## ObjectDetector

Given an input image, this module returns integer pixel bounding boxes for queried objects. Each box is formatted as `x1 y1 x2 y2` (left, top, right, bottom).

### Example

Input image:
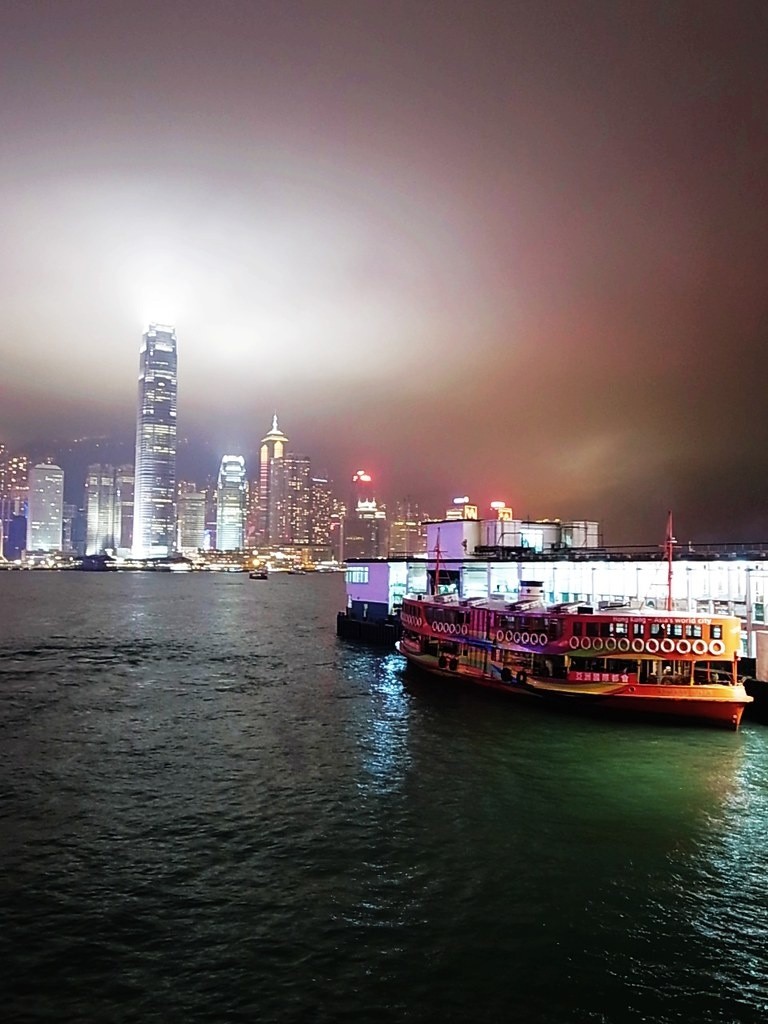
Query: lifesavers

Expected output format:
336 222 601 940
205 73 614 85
501 667 511 682
431 621 468 635
496 629 548 647
400 611 422 628
568 635 726 656
515 671 527 683
449 658 458 670
438 655 447 668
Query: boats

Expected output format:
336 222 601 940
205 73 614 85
249 570 267 580
392 509 756 733
287 567 307 575
